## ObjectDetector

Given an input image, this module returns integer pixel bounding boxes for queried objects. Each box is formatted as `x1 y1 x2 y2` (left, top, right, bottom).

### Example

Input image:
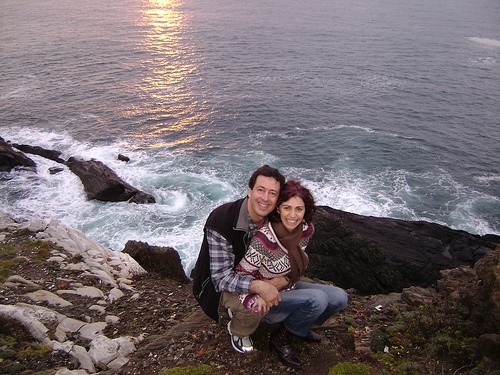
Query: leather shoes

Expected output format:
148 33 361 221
299 330 321 344
268 325 303 368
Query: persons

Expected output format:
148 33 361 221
193 165 306 354
235 181 348 368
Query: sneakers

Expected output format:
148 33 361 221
227 320 254 353
